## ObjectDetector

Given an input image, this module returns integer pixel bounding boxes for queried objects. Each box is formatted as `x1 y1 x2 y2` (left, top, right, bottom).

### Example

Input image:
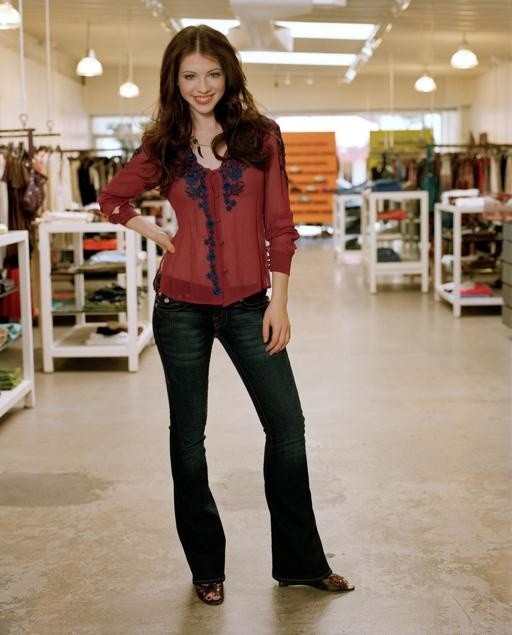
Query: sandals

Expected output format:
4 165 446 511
195 581 224 605
279 572 355 591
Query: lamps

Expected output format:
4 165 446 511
414 70 436 92
0 0 22 30
75 49 104 77
117 9 141 97
449 31 478 68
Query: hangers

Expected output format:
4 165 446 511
0 140 66 160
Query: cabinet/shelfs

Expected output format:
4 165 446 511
0 200 174 420
332 190 503 317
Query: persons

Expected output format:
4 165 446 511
96 24 356 604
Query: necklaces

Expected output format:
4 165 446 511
190 136 212 158
